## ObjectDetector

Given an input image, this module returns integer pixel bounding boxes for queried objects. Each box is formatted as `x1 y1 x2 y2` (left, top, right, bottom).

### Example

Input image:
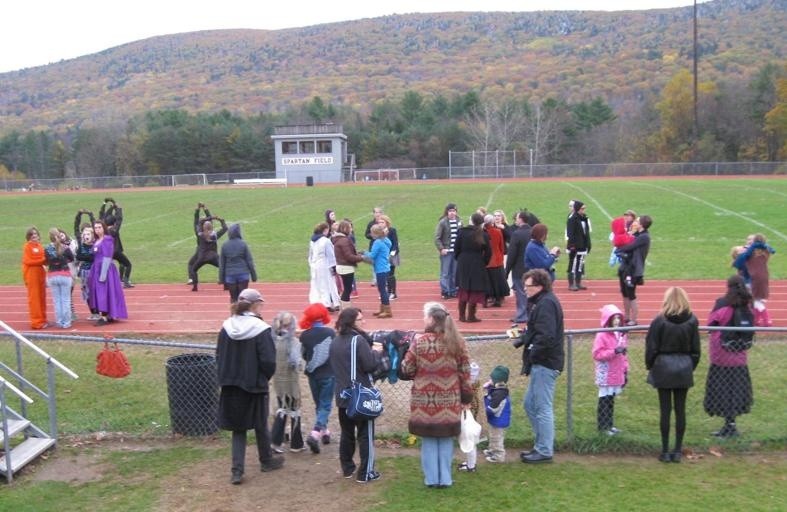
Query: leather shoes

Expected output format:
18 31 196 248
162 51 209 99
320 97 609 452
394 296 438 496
520 448 538 459
523 452 553 464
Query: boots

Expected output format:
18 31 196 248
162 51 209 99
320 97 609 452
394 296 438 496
373 304 385 316
576 271 587 290
627 297 638 326
378 304 393 318
623 297 630 323
568 272 579 291
457 300 466 322
466 303 482 322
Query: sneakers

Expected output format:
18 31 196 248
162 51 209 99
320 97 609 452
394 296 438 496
322 430 331 444
356 472 382 483
231 470 243 484
289 445 307 453
659 453 670 463
260 456 285 472
604 430 615 436
270 444 286 454
486 456 505 463
610 426 621 433
305 431 321 453
482 449 493 456
669 452 681 463
343 466 358 478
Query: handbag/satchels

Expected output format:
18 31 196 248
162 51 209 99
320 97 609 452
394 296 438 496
341 334 384 422
94 334 132 379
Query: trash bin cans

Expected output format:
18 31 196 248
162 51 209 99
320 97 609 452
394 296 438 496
164 352 221 437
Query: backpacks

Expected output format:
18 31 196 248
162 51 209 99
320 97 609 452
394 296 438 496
721 296 753 354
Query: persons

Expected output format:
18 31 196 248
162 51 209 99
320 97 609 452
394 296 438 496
483 366 512 464
592 304 630 438
369 214 398 300
360 221 392 318
74 210 97 247
328 221 344 296
566 200 592 277
492 210 512 255
22 227 52 329
504 210 533 323
481 215 510 308
341 218 358 297
58 230 80 323
88 220 127 324
523 223 561 287
703 275 755 438
434 203 463 299
215 287 285 484
187 202 216 284
330 306 383 483
365 208 384 286
610 216 636 289
644 287 701 463
330 222 364 308
454 213 492 323
520 267 565 464
745 234 772 327
103 207 134 288
218 223 257 305
614 215 653 327
43 228 73 329
270 310 307 454
190 216 228 292
104 199 126 283
618 209 638 237
458 362 480 474
509 213 518 242
400 301 473 489
732 246 752 296
307 222 341 313
298 303 337 455
477 206 488 229
75 228 103 321
567 201 589 292
325 209 336 238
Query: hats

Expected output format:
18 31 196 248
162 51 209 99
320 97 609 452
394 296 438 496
490 365 510 383
574 200 583 212
237 287 264 304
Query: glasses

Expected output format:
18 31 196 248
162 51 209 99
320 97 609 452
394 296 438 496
524 283 537 288
